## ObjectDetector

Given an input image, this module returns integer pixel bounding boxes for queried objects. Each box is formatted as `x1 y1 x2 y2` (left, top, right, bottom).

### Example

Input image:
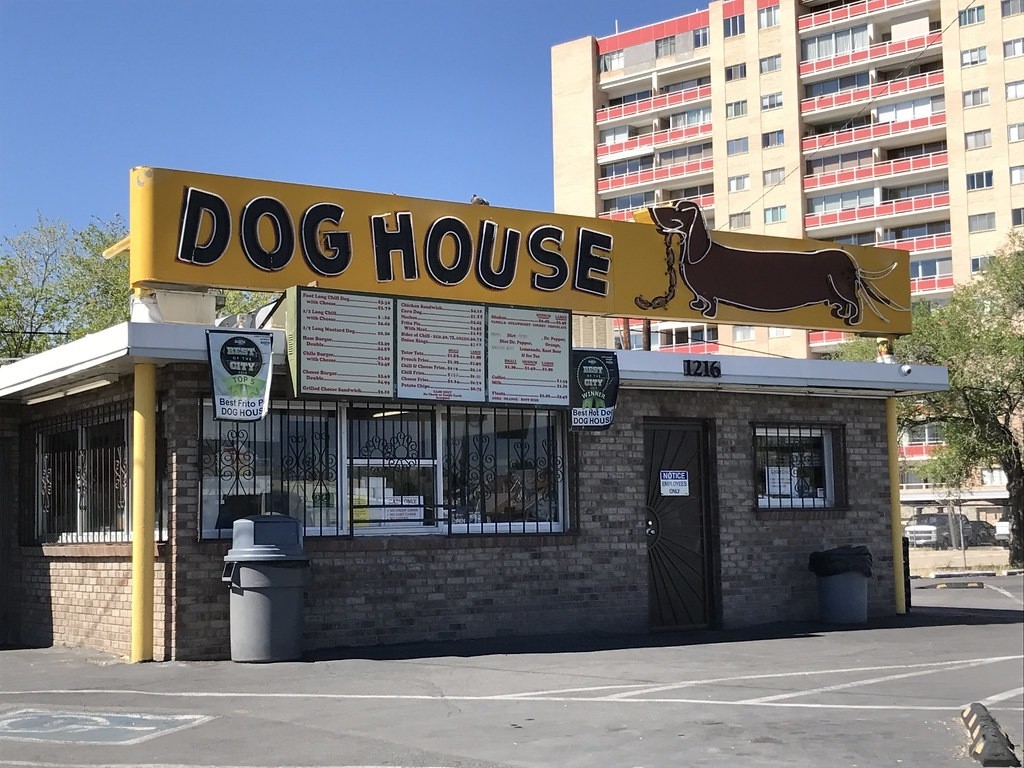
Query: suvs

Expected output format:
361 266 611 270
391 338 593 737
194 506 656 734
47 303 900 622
904 512 972 551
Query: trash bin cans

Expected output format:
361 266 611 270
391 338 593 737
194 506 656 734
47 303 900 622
223 512 312 664
808 545 875 625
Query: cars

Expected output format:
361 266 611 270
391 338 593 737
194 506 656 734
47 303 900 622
995 518 1013 546
967 521 999 546
